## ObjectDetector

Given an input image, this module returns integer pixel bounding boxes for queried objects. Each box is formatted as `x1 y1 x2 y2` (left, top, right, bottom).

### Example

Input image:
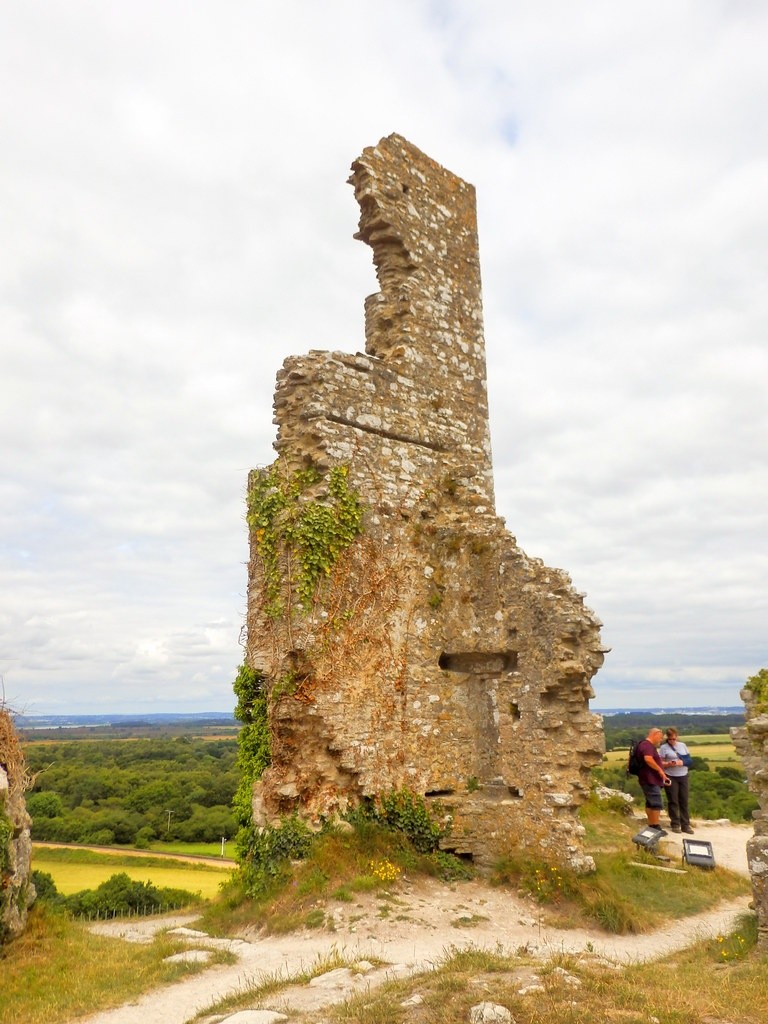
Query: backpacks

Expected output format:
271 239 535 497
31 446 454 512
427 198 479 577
626 738 649 779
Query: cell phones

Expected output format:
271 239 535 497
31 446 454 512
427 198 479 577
672 761 676 765
663 780 672 785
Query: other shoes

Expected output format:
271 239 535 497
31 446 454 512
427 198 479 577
672 825 682 833
681 824 694 834
650 824 668 836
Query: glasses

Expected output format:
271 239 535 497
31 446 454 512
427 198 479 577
669 738 676 740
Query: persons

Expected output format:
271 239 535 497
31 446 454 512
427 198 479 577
658 728 694 834
628 728 673 836
